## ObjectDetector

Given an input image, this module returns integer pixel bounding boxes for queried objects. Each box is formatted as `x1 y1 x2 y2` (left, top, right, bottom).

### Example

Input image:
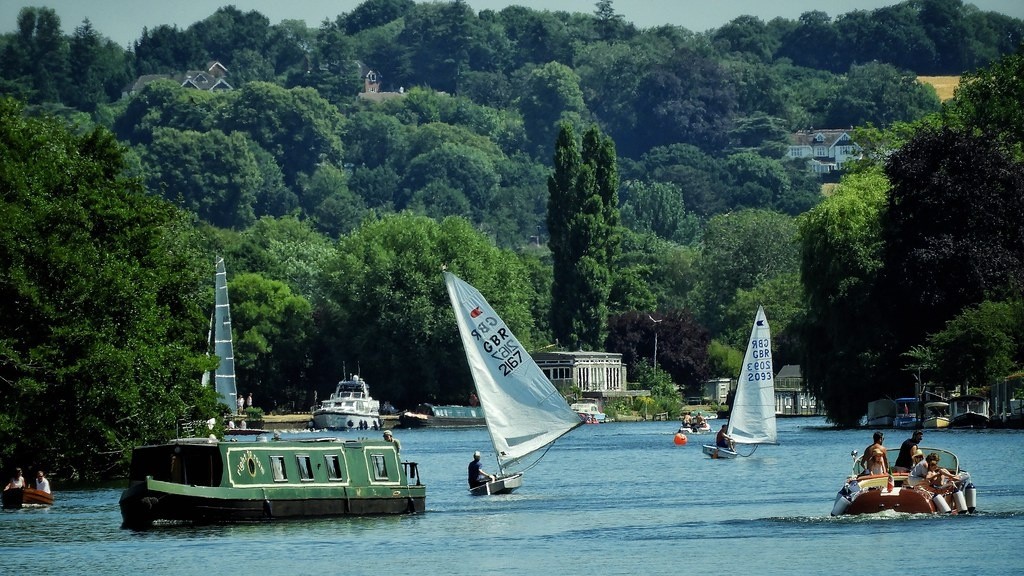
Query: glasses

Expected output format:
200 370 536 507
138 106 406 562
915 456 923 458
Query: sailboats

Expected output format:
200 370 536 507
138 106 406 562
701 305 778 460
441 263 588 495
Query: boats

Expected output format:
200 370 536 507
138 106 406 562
892 397 922 427
866 399 895 427
118 433 427 528
831 446 977 516
311 360 382 430
946 394 991 428
921 402 951 429
570 401 606 423
398 404 488 427
1 486 55 510
679 419 711 434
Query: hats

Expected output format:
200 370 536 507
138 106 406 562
872 449 883 457
383 430 392 437
911 450 926 460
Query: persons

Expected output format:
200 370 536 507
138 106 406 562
682 412 692 427
912 449 927 463
870 448 886 476
584 414 597 421
900 452 941 487
246 392 253 407
229 417 235 429
382 430 400 477
36 470 51 495
373 420 378 431
237 395 245 415
860 432 889 473
4 468 25 491
925 461 962 487
716 424 736 452
895 430 924 472
239 419 246 429
468 450 495 489
359 419 365 430
695 411 703 425
363 420 367 430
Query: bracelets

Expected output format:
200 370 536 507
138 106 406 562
486 474 491 477
